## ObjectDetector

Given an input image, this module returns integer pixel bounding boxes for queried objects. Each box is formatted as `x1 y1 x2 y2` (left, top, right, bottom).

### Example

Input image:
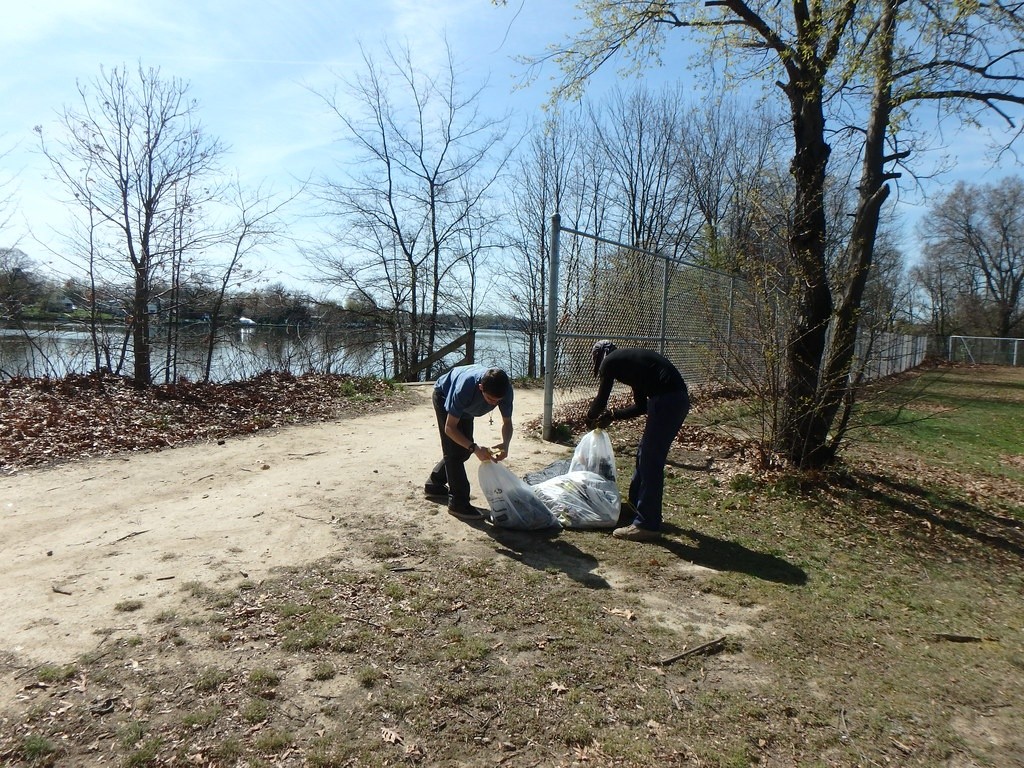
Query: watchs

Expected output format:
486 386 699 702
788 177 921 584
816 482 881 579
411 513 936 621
469 443 477 454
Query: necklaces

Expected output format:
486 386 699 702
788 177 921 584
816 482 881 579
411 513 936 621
488 410 493 425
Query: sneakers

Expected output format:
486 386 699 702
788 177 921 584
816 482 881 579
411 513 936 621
612 523 661 542
621 503 638 519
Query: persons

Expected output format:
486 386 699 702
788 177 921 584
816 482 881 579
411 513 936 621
586 340 690 540
423 364 514 519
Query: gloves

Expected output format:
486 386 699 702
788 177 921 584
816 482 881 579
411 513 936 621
586 415 596 430
597 408 615 429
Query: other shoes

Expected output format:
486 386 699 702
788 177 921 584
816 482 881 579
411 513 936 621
447 501 484 520
424 483 448 498
